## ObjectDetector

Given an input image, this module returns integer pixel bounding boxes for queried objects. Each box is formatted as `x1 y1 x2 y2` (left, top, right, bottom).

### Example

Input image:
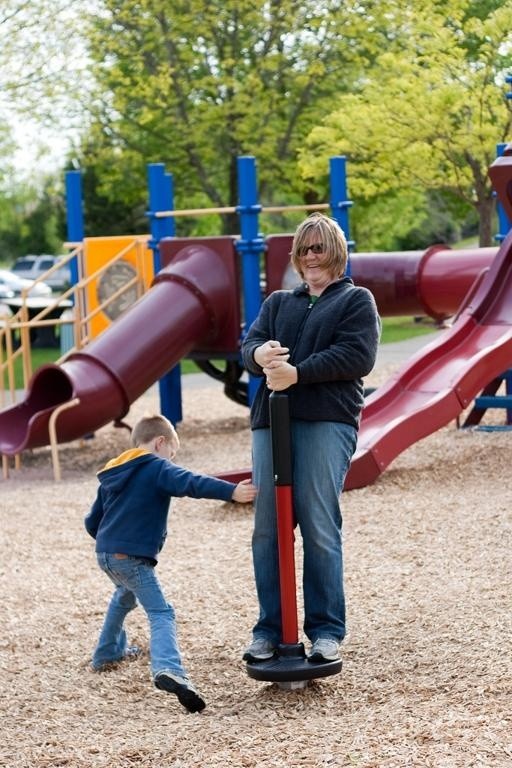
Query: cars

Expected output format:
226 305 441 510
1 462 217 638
0 256 72 348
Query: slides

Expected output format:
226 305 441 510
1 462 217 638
0 244 235 457
208 227 511 495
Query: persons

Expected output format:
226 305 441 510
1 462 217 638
241 211 381 663
85 414 258 713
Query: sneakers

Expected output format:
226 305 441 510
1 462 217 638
91 646 143 672
307 637 341 661
242 638 278 660
155 672 206 713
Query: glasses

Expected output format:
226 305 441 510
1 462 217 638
297 243 324 256
